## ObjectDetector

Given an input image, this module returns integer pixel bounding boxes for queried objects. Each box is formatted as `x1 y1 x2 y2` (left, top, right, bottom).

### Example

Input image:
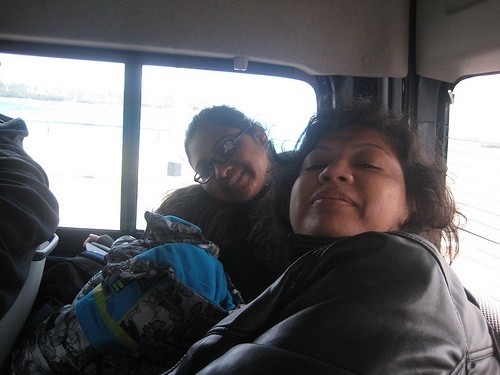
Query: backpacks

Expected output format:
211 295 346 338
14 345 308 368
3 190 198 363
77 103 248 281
7 209 247 375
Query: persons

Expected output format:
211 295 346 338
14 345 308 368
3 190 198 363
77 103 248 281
82 106 306 305
0 114 59 322
166 91 500 375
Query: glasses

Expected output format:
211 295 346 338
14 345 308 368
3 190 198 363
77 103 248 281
193 125 252 185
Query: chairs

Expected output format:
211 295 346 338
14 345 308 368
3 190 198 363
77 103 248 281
0 232 58 364
